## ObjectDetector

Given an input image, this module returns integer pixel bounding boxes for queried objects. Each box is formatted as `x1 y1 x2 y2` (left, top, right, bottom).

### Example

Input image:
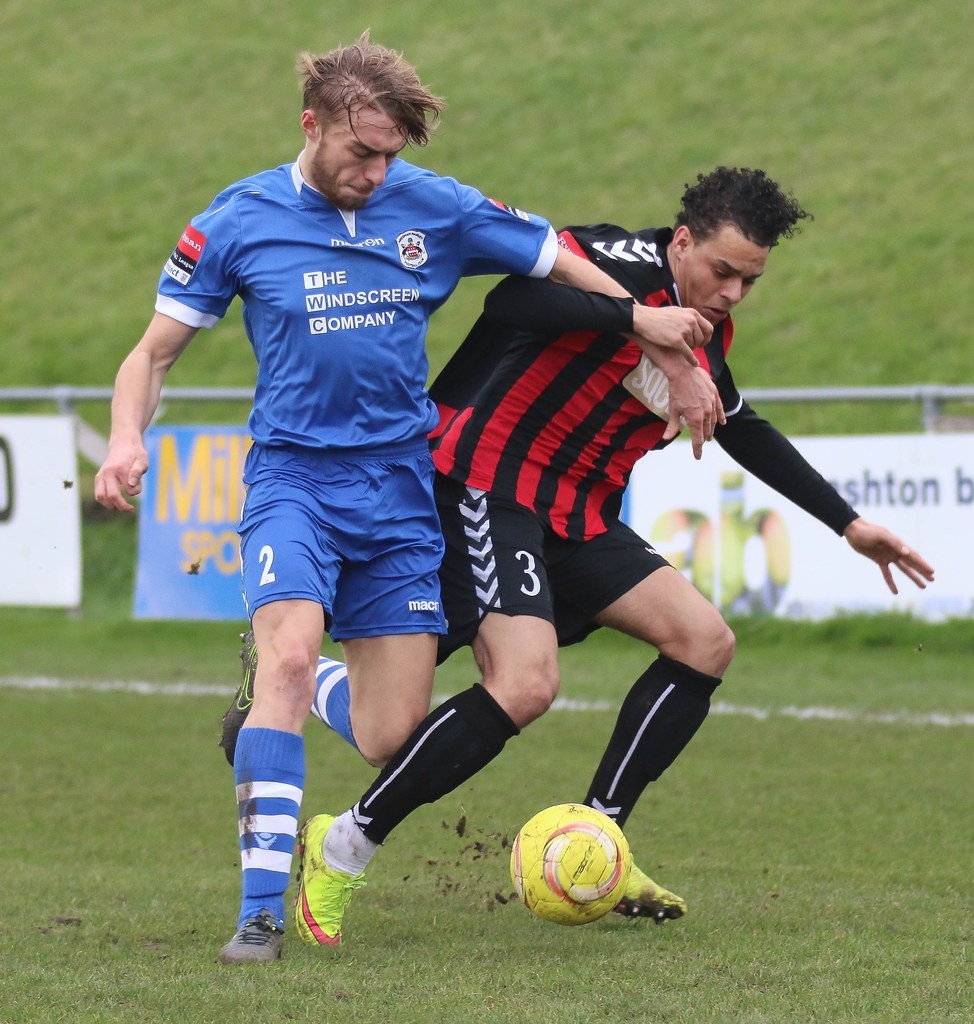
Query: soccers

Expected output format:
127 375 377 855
509 803 632 927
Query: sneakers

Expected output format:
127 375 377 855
295 814 366 947
614 861 688 924
217 630 257 766
219 908 286 965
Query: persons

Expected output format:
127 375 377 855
292 167 939 953
92 40 728 971
